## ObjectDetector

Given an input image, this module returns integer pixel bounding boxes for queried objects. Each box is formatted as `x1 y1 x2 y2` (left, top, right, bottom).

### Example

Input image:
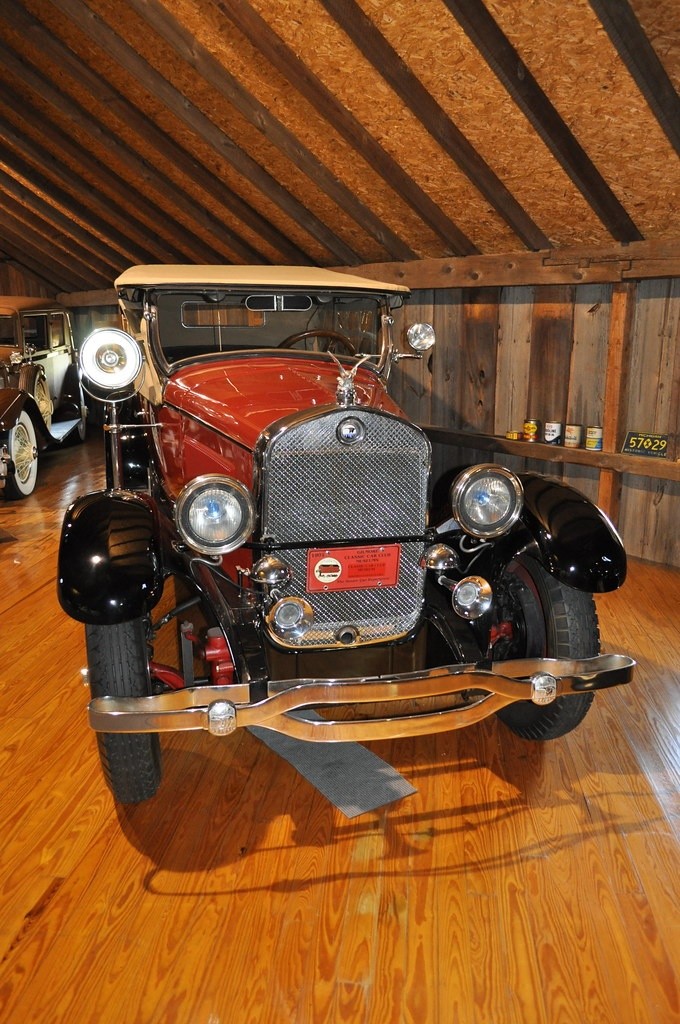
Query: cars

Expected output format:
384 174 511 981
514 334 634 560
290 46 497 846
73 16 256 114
0 296 90 500
57 263 639 805
